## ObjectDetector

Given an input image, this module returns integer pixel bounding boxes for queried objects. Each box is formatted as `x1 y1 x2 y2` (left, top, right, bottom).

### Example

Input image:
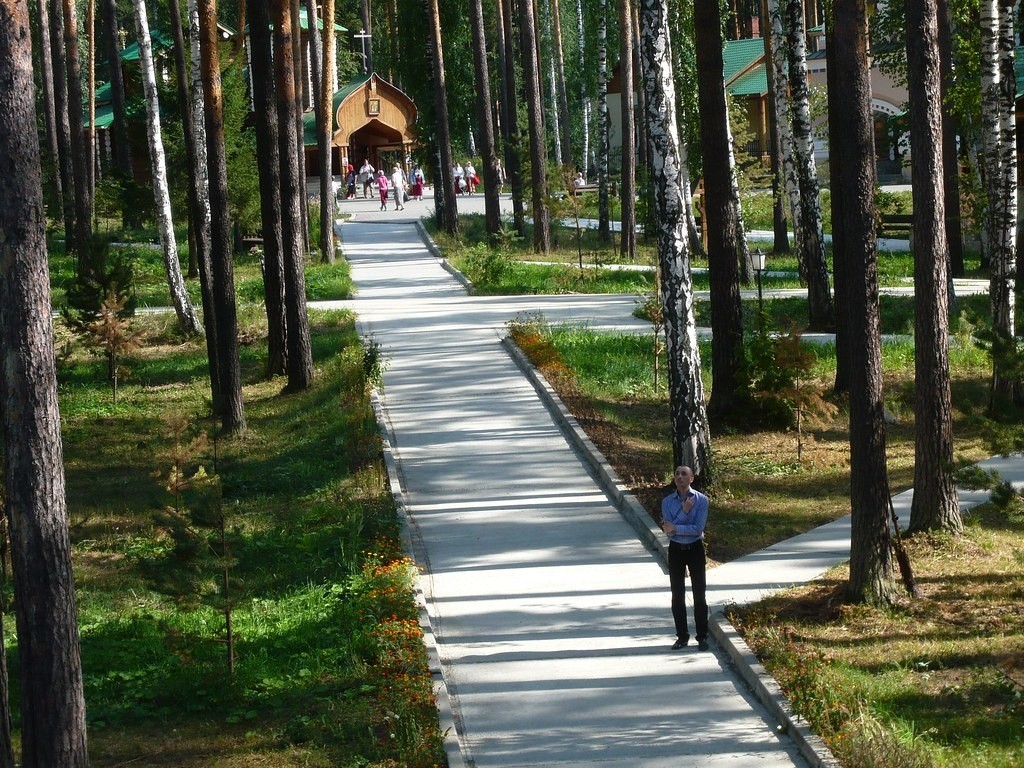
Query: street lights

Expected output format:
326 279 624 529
750 244 769 339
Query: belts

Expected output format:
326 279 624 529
670 538 702 551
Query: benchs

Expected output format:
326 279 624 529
562 182 617 201
876 213 914 240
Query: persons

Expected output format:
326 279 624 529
332 176 340 212
593 173 601 184
359 159 376 198
561 172 586 200
661 464 710 650
464 161 476 196
452 162 465 196
375 170 389 211
346 165 357 199
494 159 506 195
391 162 407 211
411 164 425 201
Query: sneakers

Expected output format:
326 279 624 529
671 638 688 649
699 638 709 651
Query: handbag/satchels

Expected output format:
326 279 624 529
385 191 388 198
458 177 467 189
472 175 480 184
404 192 410 202
367 177 374 183
